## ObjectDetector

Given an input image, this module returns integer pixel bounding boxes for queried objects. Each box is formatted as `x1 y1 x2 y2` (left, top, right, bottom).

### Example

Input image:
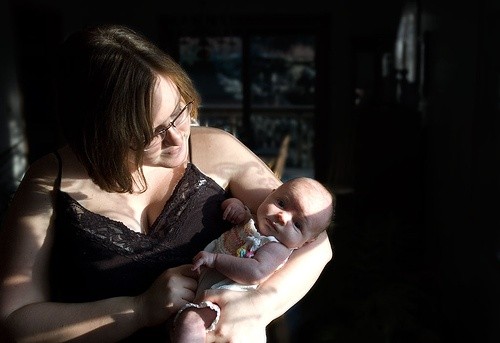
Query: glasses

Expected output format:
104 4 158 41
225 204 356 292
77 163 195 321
128 82 196 152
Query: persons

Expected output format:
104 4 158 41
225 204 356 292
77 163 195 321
0 27 333 343
170 177 336 343
